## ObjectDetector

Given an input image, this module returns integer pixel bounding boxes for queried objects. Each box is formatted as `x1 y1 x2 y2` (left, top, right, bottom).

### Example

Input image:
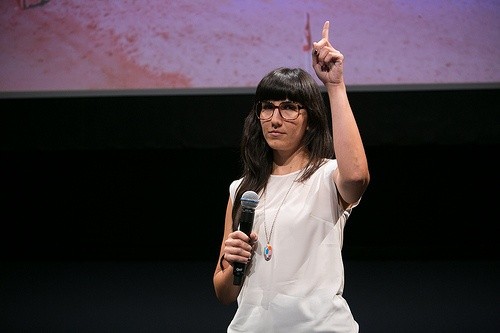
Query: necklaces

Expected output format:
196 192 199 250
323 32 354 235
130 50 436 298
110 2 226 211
263 171 301 261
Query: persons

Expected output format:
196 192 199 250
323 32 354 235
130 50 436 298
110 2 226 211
213 21 371 333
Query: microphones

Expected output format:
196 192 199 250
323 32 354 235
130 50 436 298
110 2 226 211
233 191 260 286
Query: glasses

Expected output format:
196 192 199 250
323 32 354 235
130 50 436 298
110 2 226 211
256 100 305 120
